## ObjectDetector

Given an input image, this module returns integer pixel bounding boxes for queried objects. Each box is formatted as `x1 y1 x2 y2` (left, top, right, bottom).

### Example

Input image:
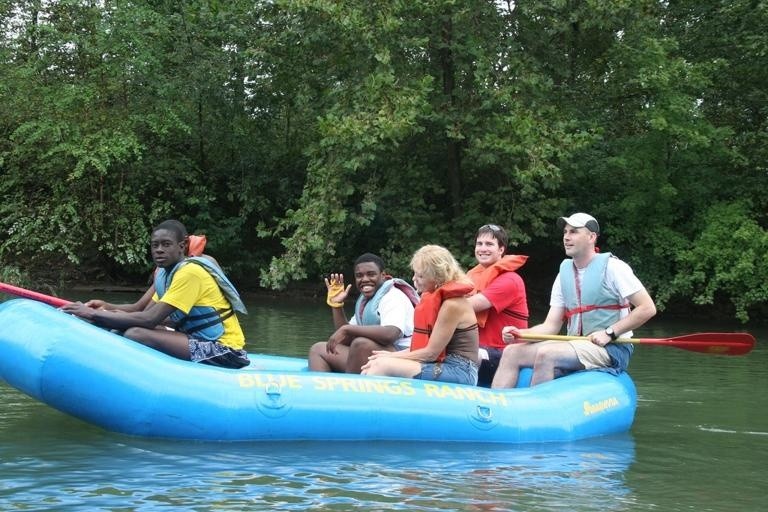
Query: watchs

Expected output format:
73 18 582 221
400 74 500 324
604 325 618 341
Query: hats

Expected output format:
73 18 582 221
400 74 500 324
557 212 600 236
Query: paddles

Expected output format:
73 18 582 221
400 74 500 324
507 333 754 354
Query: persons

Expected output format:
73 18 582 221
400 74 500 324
61 219 251 369
307 252 421 374
360 243 480 385
414 223 530 386
490 212 657 390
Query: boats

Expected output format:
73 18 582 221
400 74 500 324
0 297 638 446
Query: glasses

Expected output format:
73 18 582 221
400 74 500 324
479 225 501 232
